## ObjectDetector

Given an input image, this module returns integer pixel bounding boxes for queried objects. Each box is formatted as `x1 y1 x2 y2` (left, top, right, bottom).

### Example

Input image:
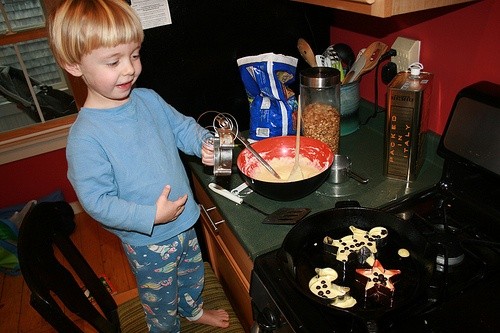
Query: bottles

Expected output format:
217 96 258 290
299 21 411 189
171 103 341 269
298 66 341 155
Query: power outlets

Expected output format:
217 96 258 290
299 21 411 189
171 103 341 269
391 35 421 73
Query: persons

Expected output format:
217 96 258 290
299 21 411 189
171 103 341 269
46 0 229 333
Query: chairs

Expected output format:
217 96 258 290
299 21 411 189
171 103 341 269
16 199 246 333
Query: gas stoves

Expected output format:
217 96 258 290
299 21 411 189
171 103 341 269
248 82 500 333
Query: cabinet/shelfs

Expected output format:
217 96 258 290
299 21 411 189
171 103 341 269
192 171 252 333
293 0 473 17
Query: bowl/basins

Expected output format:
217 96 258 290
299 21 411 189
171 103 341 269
236 135 335 202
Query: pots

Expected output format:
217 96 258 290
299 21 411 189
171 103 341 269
279 200 438 324
